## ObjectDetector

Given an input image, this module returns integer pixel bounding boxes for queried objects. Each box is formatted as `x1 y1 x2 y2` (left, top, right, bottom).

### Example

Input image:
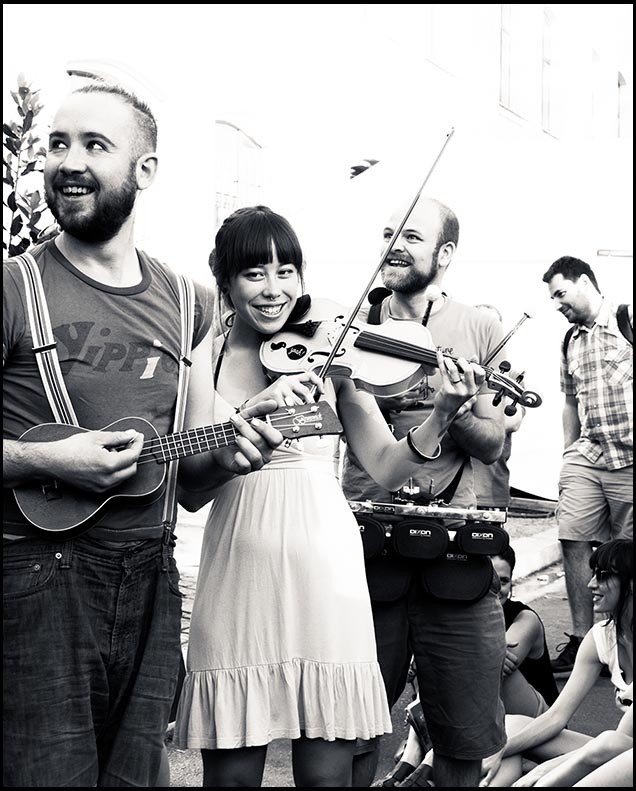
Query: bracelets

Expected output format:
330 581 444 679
407 425 442 462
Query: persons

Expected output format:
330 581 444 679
0 83 283 788
333 198 633 788
178 206 478 787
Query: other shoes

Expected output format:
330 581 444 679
550 632 585 679
367 761 436 789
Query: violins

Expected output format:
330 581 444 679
258 293 543 416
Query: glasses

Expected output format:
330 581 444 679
592 566 617 581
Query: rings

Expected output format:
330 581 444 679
453 378 462 383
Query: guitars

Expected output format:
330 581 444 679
12 400 344 533
